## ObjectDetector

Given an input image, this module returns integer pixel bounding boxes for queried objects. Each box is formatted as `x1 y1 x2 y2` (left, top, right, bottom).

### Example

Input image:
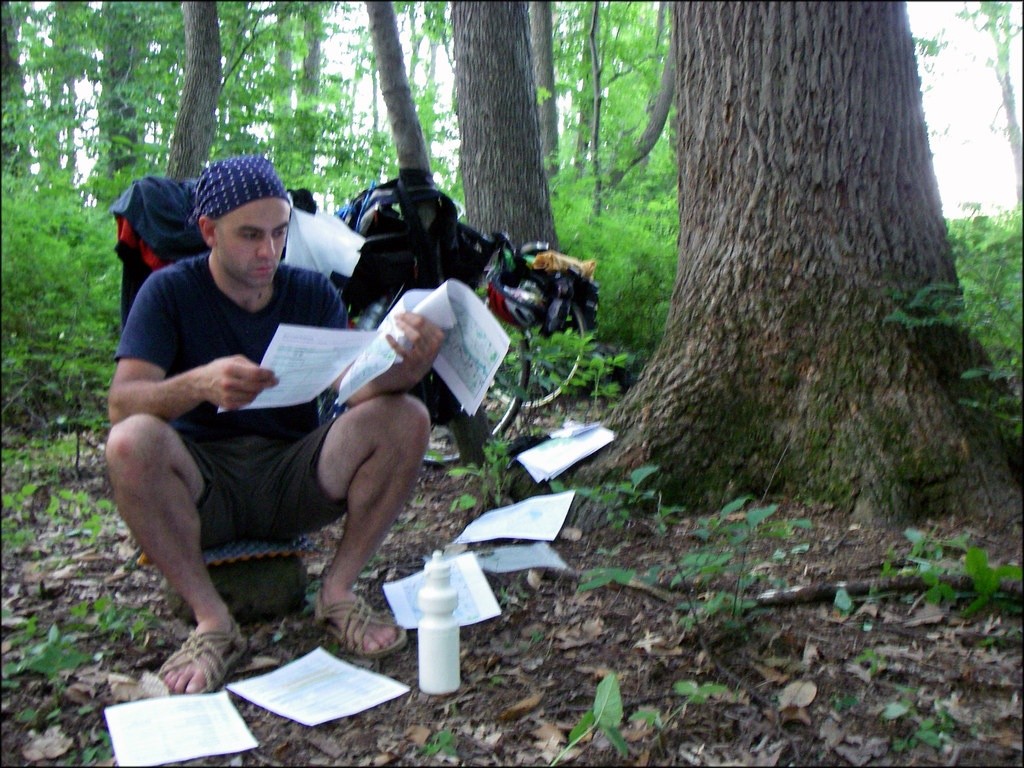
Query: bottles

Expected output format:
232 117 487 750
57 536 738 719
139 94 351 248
355 296 386 330
449 400 493 467
416 548 460 694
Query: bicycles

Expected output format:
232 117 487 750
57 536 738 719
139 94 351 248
315 231 590 468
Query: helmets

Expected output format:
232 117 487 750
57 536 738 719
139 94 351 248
486 265 543 328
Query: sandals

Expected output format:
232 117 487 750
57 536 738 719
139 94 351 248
158 615 241 695
313 584 407 658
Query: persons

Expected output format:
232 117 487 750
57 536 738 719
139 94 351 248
104 156 448 699
